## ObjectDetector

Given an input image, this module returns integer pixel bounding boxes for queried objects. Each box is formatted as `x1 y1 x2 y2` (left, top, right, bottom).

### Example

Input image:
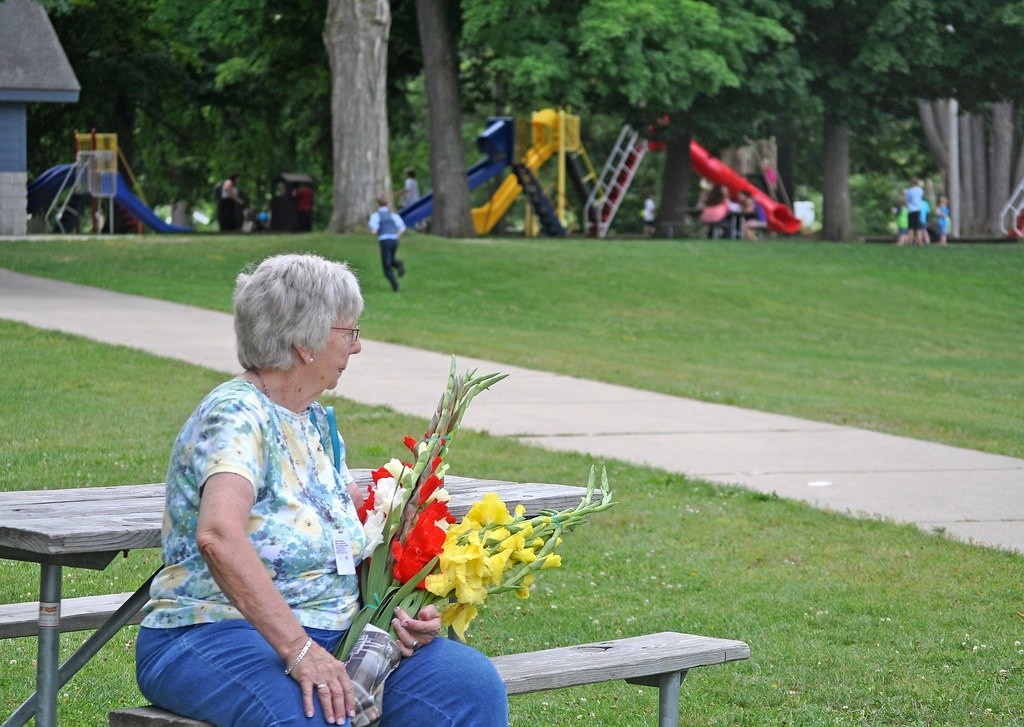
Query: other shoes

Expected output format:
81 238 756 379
395 259 404 277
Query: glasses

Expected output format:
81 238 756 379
330 324 361 342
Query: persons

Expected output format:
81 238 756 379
699 183 768 238
367 196 406 291
893 176 953 246
216 172 249 231
644 194 657 235
394 169 426 232
133 253 508 727
292 183 314 232
244 204 269 231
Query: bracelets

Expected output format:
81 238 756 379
284 637 312 674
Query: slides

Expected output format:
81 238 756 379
472 144 553 235
398 159 506 228
655 126 802 233
103 167 191 234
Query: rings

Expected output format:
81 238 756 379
410 640 418 649
317 684 326 689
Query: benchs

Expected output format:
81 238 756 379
0 590 152 642
702 220 765 231
109 631 751 727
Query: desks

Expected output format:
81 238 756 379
674 209 751 239
0 470 607 727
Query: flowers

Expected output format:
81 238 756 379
350 364 611 645
